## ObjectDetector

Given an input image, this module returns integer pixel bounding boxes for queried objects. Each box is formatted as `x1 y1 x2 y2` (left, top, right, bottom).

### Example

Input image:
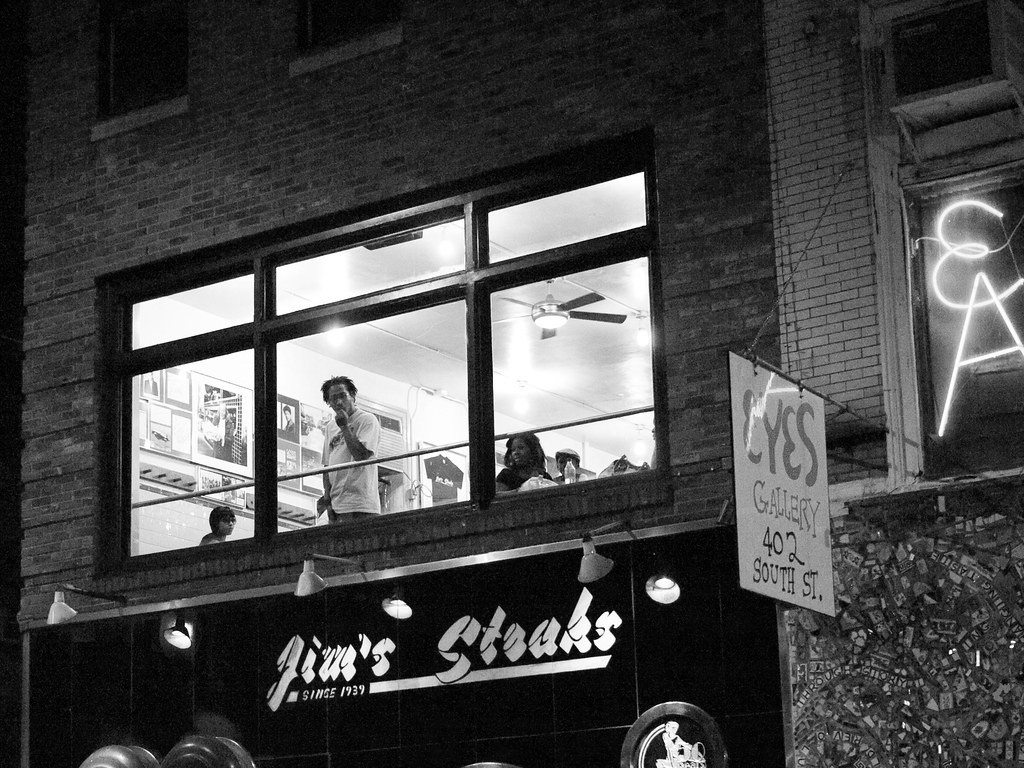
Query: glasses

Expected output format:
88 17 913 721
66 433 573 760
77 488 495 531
330 391 351 401
222 516 237 525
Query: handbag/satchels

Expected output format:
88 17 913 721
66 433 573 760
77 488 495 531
598 455 650 478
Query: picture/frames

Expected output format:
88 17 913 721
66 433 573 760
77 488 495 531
138 368 332 510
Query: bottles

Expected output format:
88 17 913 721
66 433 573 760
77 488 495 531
564 458 576 484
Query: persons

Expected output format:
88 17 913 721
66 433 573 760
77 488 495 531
199 505 237 546
495 431 553 494
281 406 295 433
551 448 587 484
144 371 159 396
650 416 657 470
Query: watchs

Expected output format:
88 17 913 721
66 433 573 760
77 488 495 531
319 374 381 524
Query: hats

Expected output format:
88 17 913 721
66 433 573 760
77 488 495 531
555 448 580 461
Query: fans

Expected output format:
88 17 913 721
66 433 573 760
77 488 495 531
489 292 628 340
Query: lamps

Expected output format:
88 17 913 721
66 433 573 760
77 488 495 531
380 582 414 620
46 583 129 626
162 608 192 649
293 551 376 597
577 517 631 583
645 550 685 606
